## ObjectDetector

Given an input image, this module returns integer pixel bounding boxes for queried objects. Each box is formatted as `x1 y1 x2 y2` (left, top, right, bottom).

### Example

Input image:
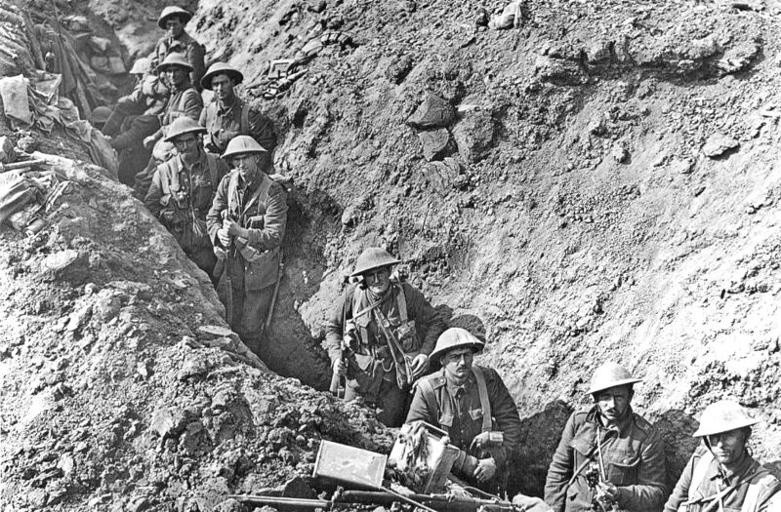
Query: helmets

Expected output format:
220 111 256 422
157 6 192 30
582 360 644 396
428 327 486 361
218 135 269 160
692 398 761 442
348 247 403 279
200 62 244 90
129 58 155 75
162 117 207 143
155 52 195 75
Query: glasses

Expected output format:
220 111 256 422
709 432 748 445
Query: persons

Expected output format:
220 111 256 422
664 396 781 512
539 359 667 511
403 325 524 495
319 244 446 427
155 3 205 79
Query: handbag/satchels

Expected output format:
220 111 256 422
477 448 511 491
396 350 440 389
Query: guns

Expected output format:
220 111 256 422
230 485 523 511
